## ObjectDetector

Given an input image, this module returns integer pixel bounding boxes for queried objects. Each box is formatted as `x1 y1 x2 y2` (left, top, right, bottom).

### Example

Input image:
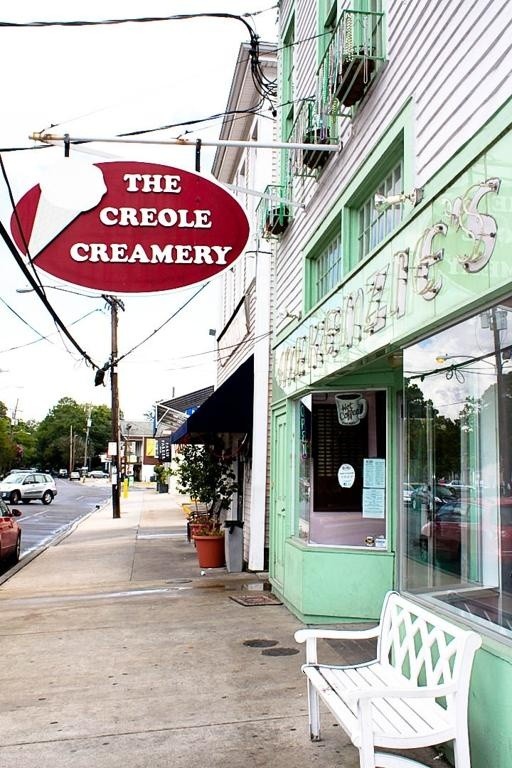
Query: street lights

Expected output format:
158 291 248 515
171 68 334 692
435 355 512 479
16 285 122 518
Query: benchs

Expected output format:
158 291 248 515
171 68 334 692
294 593 484 765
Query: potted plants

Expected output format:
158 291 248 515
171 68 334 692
153 437 241 567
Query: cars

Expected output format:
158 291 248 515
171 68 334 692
88 470 110 479
403 479 512 563
0 496 22 563
0 468 58 505
59 469 80 481
